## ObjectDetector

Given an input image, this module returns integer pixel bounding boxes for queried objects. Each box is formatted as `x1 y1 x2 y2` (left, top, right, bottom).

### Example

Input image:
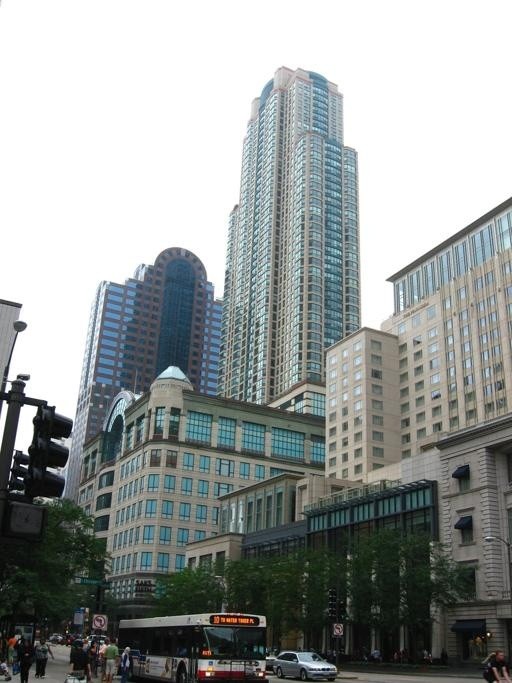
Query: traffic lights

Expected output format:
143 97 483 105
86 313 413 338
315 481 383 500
24 403 75 498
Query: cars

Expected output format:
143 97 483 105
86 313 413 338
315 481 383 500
272 650 338 682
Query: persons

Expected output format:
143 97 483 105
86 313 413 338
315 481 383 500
265 647 433 664
67 639 131 683
483 650 512 683
0 631 54 683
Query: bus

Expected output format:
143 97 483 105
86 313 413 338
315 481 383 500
116 611 270 683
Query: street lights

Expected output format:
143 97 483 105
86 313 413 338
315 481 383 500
484 536 512 595
